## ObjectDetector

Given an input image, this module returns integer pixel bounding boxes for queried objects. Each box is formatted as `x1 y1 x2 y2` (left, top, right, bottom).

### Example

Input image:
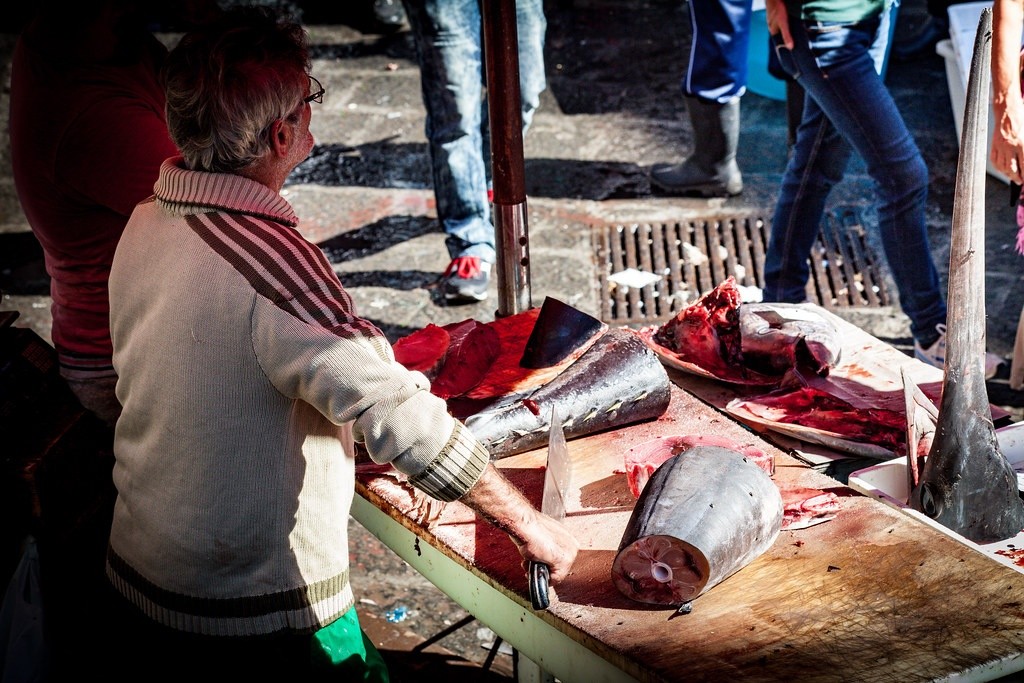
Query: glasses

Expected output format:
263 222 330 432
299 75 324 107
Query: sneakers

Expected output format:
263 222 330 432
914 323 1008 382
442 255 492 301
486 189 496 227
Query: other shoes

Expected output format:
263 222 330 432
983 334 1015 360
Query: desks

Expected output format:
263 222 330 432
350 370 1024 682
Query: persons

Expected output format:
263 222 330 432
650 0 1023 389
97 8 579 683
404 0 548 304
8 1 185 436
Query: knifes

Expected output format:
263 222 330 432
528 404 573 611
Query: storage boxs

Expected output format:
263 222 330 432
849 418 1024 575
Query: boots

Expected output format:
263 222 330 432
785 80 805 158
647 97 742 195
